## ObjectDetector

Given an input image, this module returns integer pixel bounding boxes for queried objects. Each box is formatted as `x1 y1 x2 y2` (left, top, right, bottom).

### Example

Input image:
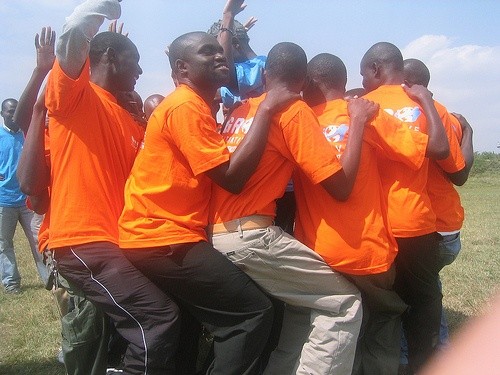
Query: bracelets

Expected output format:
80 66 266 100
217 25 234 37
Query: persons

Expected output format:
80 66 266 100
143 94 166 120
293 52 450 374
44 1 184 374
117 33 275 375
119 92 144 121
346 88 368 100
207 0 299 373
397 58 469 374
16 84 117 373
1 98 51 290
209 41 380 375
17 26 56 151
355 42 475 374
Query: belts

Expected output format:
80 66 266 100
205 215 275 235
